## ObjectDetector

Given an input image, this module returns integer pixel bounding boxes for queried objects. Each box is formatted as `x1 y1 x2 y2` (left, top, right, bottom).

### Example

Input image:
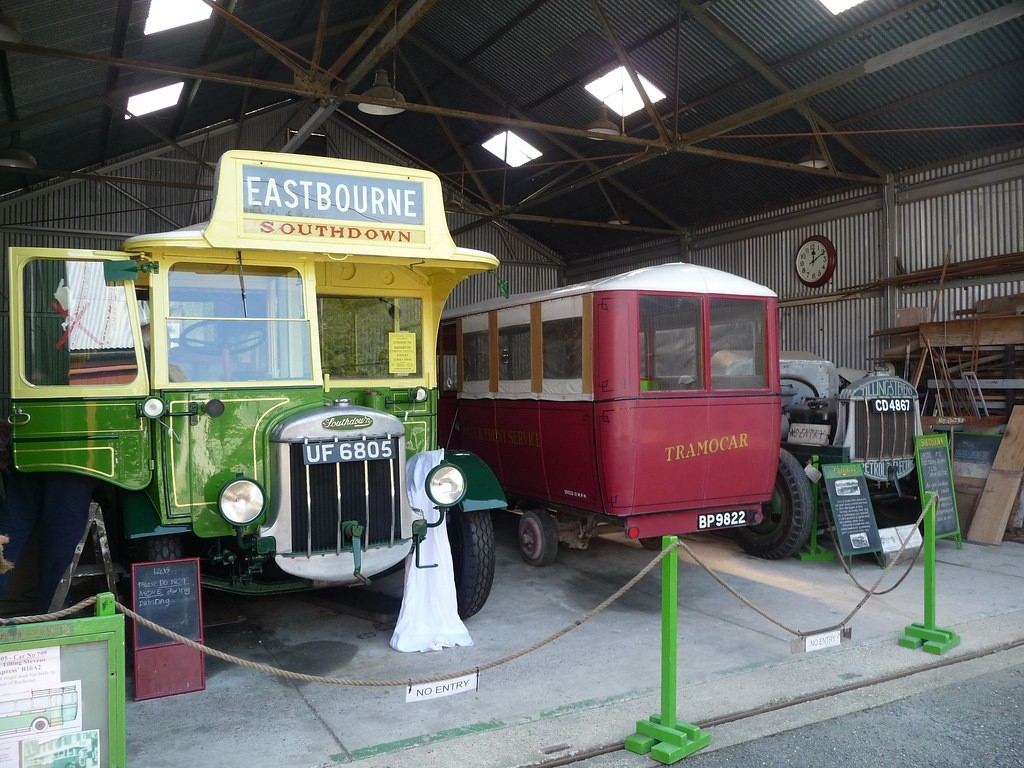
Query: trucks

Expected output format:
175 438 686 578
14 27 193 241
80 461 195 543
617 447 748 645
690 348 926 562
2 149 511 622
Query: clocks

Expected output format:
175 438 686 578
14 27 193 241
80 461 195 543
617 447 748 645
794 235 837 288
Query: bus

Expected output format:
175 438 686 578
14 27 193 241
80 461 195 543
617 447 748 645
437 259 783 568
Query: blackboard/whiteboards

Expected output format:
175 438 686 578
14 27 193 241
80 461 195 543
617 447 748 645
822 477 890 556
914 448 967 538
131 557 204 654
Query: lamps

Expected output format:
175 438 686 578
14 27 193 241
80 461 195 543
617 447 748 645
0 147 38 169
357 43 405 116
798 138 828 169
583 103 620 141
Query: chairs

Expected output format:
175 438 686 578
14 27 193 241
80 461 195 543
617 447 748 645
170 346 238 382
866 307 929 383
